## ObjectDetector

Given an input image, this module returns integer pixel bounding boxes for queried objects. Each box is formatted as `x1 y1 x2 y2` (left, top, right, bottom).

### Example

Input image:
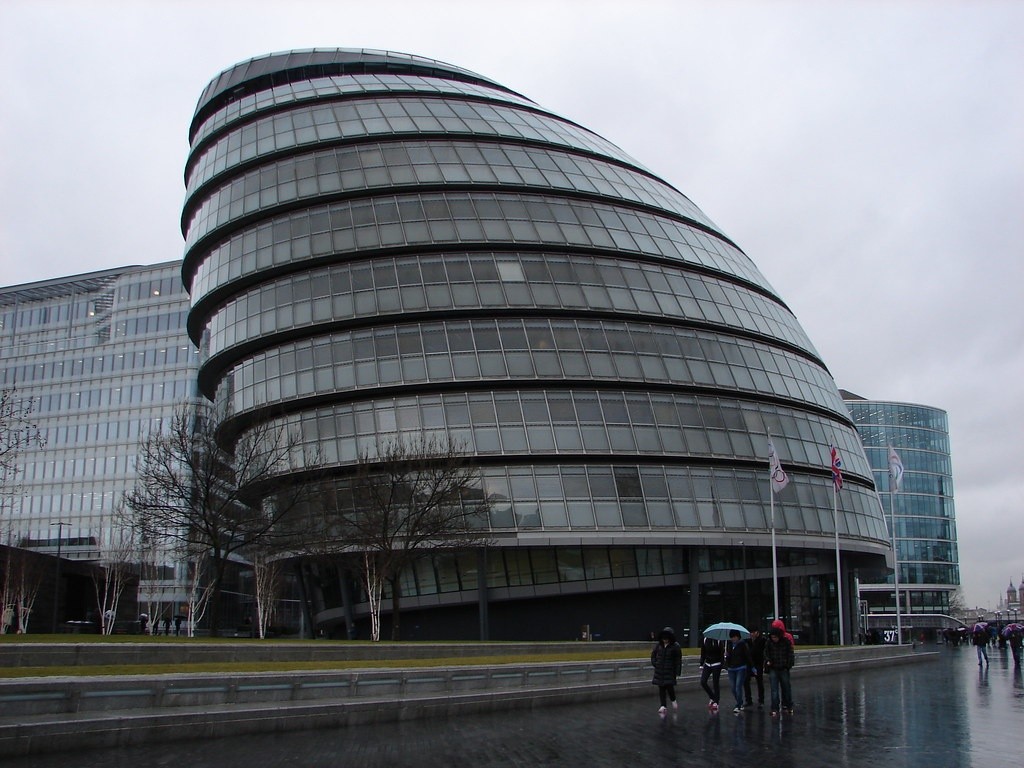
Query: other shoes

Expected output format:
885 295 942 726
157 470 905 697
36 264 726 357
740 706 744 710
772 711 776 717
788 708 793 713
707 699 714 706
733 707 740 712
711 702 719 713
671 699 677 712
658 705 668 713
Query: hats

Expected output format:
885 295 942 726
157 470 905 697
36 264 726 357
748 623 761 632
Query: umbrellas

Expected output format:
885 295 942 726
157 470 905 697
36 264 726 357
704 622 750 660
970 622 987 633
1001 623 1024 638
174 614 186 618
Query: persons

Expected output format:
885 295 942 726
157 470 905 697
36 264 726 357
652 628 683 713
140 614 182 637
859 622 1024 665
699 620 797 717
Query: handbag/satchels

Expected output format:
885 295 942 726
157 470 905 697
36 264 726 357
651 650 657 667
763 660 769 674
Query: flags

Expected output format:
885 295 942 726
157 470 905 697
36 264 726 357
888 447 904 494
768 435 789 494
830 446 843 491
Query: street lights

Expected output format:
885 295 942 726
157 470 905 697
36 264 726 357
50 521 73 635
